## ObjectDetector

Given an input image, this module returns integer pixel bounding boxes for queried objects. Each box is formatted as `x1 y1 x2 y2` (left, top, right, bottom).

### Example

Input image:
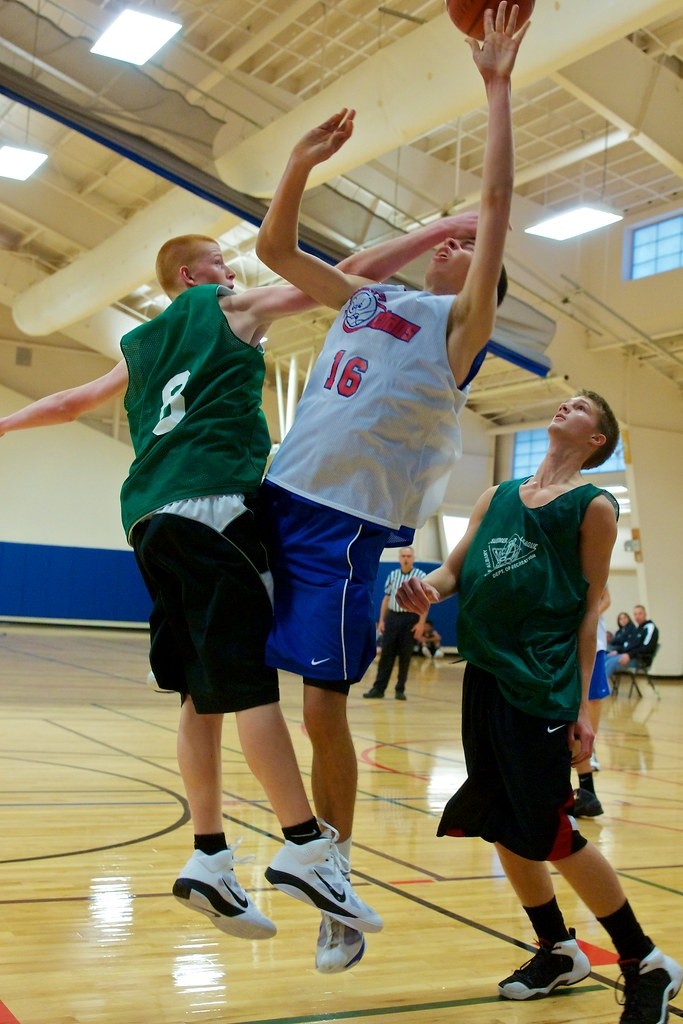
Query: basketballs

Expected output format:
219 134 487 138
445 0 535 41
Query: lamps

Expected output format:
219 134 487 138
0 0 47 181
90 0 182 66
523 119 625 242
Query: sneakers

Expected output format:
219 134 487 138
566 794 604 818
316 910 365 973
615 938 683 1024
171 837 276 940
264 814 383 933
497 929 591 999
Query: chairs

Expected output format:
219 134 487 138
609 644 659 699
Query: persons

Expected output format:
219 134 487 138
148 0 534 975
363 547 659 817
394 389 683 1024
0 209 513 939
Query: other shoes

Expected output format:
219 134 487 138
363 688 384 698
395 693 406 700
